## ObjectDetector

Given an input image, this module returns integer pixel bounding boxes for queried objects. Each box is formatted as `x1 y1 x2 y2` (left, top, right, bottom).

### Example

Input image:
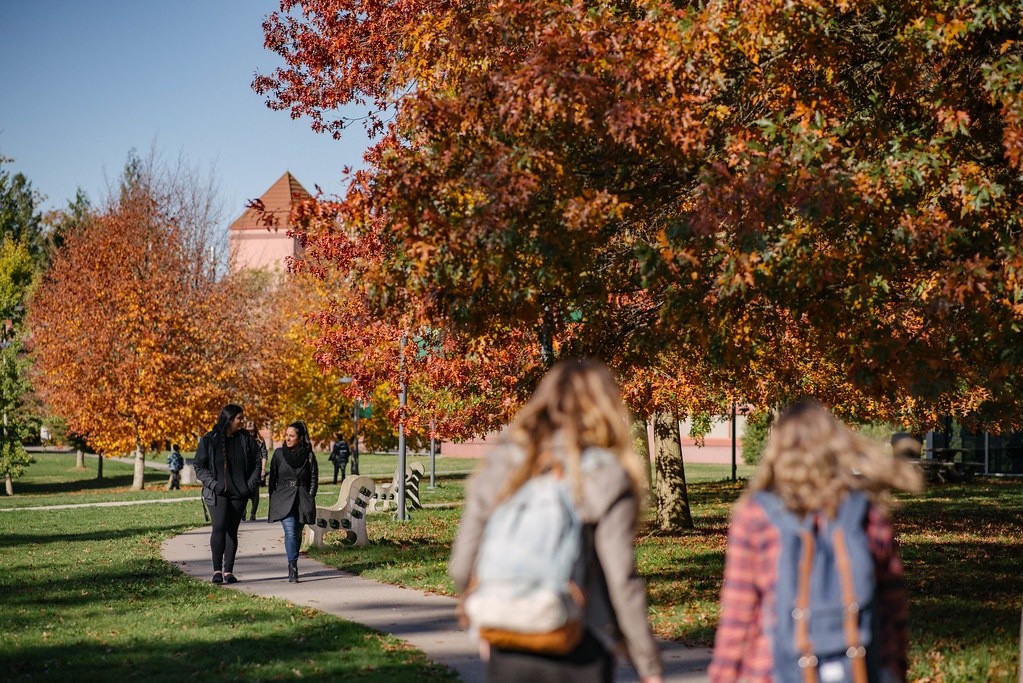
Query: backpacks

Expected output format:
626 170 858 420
463 439 618 653
752 486 881 683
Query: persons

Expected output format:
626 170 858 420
704 396 926 683
241 420 268 522
447 356 666 683
167 444 184 491
327 432 352 485
267 421 319 586
192 403 263 585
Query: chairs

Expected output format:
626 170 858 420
302 461 426 550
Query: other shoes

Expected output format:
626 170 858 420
288 561 298 582
242 513 256 521
224 574 237 583
212 573 223 582
